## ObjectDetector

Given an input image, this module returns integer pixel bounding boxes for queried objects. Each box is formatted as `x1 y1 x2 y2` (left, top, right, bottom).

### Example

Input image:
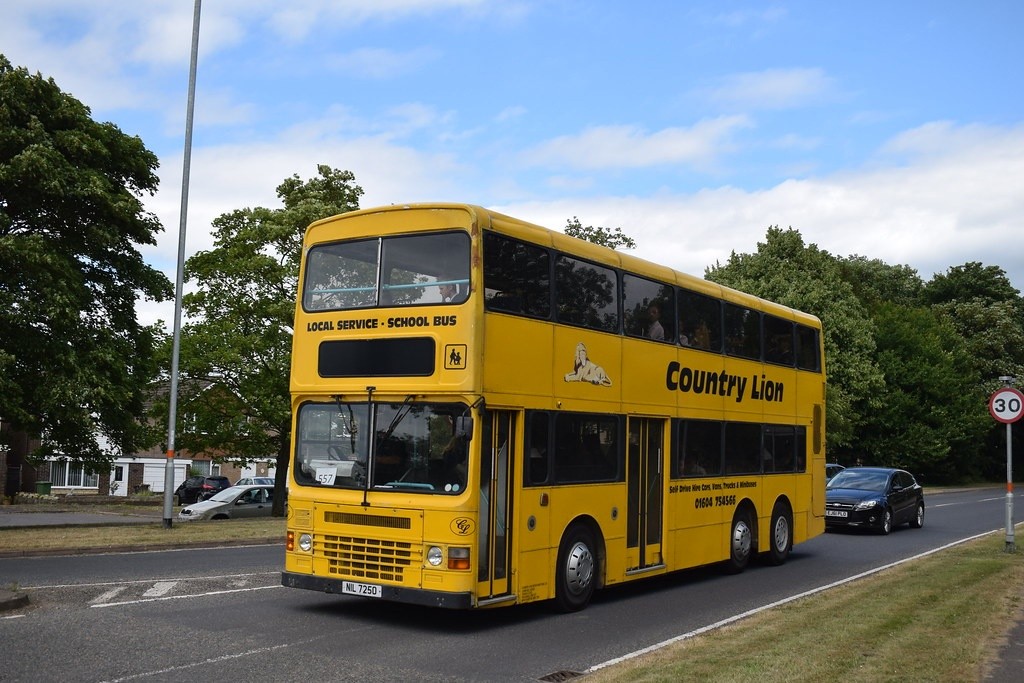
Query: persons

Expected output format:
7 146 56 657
444 432 803 491
435 273 468 304
852 458 864 466
621 304 788 363
350 416 408 489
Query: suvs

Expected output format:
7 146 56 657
174 474 233 506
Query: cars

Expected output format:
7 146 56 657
233 476 288 501
177 484 287 522
823 465 926 535
826 462 847 487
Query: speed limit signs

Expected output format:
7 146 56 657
989 388 1024 423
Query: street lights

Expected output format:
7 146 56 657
998 375 1015 554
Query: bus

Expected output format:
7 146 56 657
280 199 828 612
